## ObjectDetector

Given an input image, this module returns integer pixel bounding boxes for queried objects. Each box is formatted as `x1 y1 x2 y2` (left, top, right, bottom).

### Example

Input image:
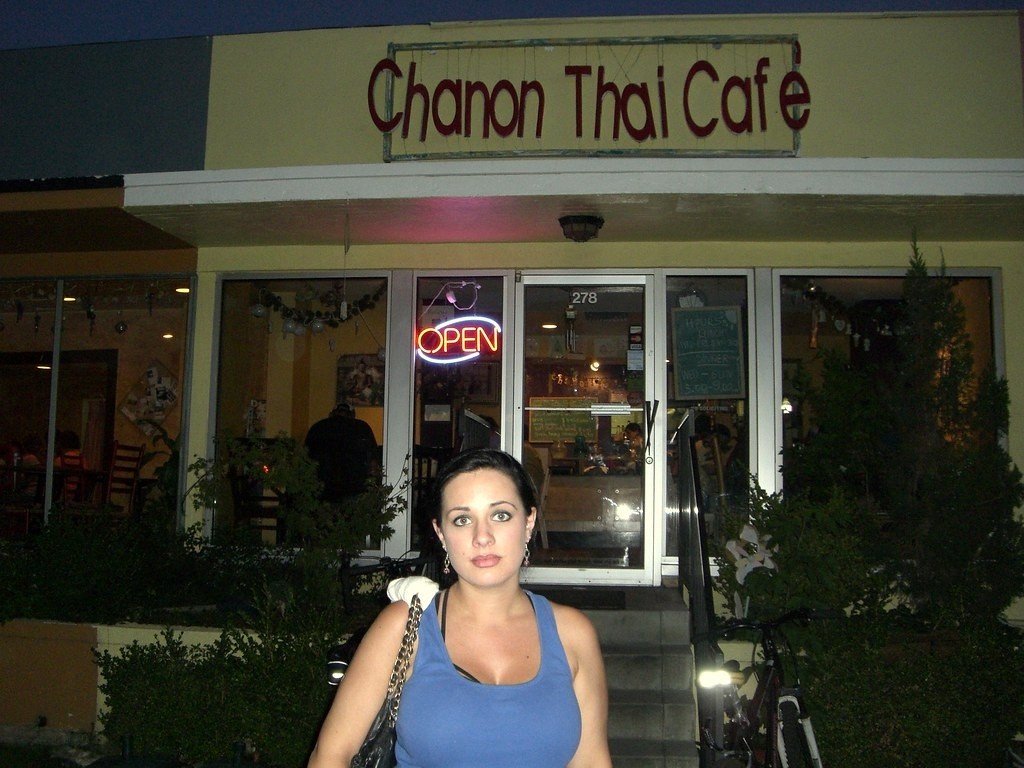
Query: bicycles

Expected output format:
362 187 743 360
690 605 825 768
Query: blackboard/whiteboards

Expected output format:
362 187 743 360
529 396 599 444
669 304 747 401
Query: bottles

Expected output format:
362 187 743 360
615 420 632 449
550 440 568 458
573 431 603 460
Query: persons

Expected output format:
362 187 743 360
306 403 379 501
615 415 732 502
0 429 89 506
307 447 614 768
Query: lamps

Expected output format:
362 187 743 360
557 215 605 243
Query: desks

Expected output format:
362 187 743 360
9 465 110 509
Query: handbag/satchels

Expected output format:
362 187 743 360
351 595 422 768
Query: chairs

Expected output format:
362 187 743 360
228 437 296 551
61 439 147 529
0 450 43 539
535 468 551 556
